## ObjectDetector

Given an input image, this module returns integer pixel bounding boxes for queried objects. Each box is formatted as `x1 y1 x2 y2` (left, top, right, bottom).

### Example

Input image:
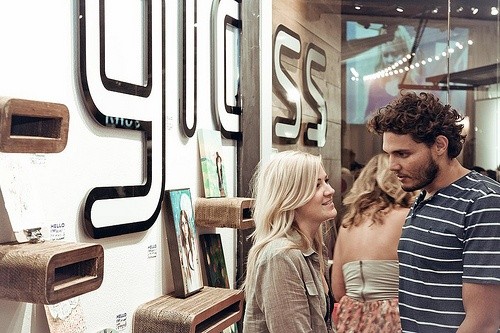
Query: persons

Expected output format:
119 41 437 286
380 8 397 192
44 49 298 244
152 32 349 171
365 90 500 333
215 152 226 196
321 154 415 332
174 210 204 292
235 152 338 333
